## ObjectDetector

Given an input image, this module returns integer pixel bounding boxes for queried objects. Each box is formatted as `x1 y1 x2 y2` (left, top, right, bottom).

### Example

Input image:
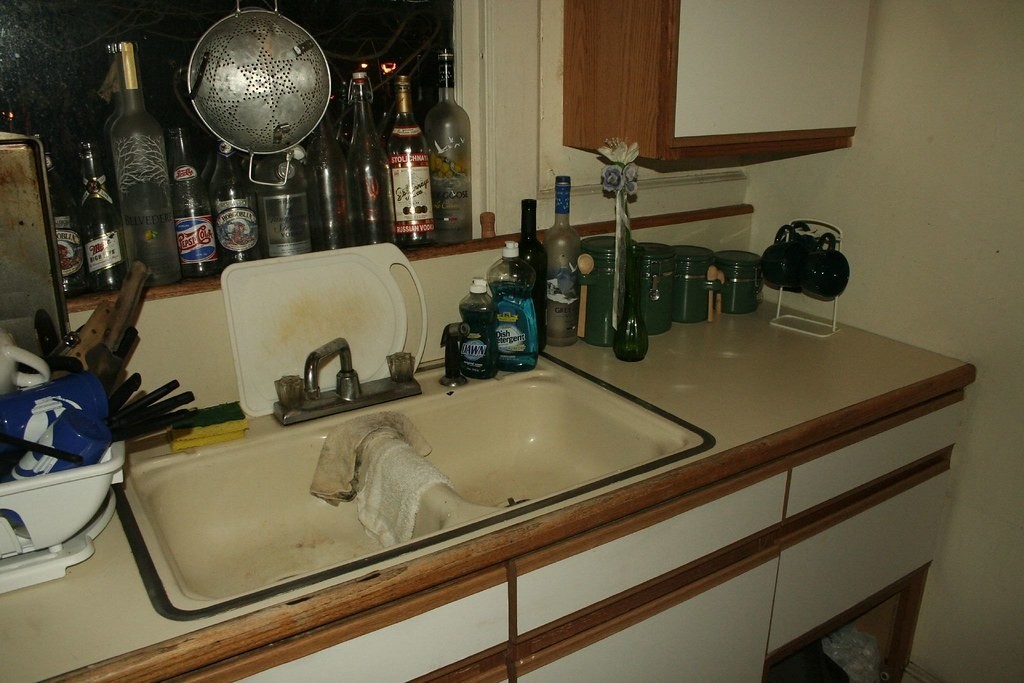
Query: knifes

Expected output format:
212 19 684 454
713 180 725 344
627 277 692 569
63 260 151 384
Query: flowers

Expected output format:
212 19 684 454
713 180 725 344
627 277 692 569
597 138 640 331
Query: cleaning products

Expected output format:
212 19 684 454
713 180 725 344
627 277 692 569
458 276 499 380
485 240 539 373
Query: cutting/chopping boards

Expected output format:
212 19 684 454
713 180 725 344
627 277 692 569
221 242 428 418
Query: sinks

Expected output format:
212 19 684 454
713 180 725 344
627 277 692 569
371 352 706 515
119 412 457 613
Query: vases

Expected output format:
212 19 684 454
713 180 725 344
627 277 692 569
614 240 649 361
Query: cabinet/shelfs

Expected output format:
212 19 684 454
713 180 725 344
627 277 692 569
514 544 781 683
564 0 870 160
763 459 950 683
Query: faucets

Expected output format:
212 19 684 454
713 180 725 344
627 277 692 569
272 337 423 427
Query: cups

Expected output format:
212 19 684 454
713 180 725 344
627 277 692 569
0 329 51 394
3 407 112 524
762 221 850 298
0 370 109 451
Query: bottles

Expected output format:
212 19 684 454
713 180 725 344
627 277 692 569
110 41 182 287
386 75 437 249
509 199 548 352
77 138 127 291
32 134 89 298
170 128 219 277
426 48 473 244
255 152 312 258
211 136 261 271
544 175 582 347
307 109 362 252
346 72 392 249
